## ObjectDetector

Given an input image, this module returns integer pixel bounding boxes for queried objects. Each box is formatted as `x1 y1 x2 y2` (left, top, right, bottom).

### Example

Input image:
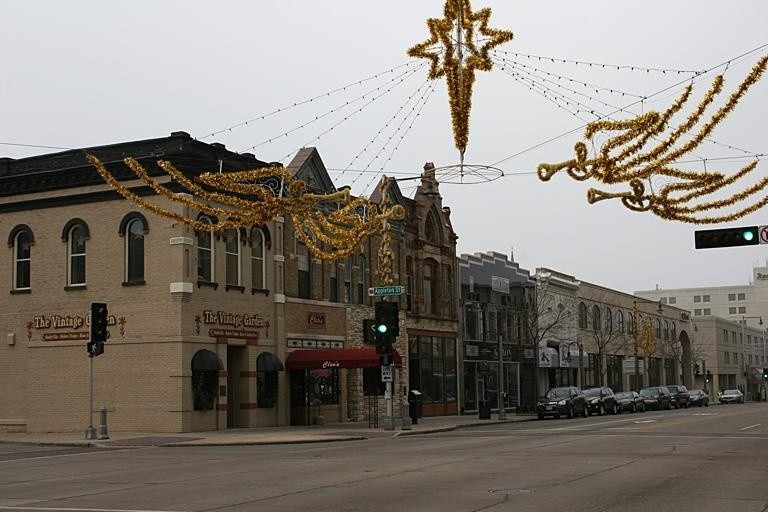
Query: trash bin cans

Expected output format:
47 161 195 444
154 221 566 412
478 400 491 419
408 389 423 424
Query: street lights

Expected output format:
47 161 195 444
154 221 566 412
742 314 765 398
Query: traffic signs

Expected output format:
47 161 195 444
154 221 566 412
367 286 405 297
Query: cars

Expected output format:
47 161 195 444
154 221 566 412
718 389 745 405
533 384 711 421
482 389 519 408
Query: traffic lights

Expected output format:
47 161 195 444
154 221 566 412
693 225 759 250
705 370 711 380
763 368 768 381
373 300 400 356
695 364 701 374
706 379 710 383
85 341 105 356
91 301 109 343
